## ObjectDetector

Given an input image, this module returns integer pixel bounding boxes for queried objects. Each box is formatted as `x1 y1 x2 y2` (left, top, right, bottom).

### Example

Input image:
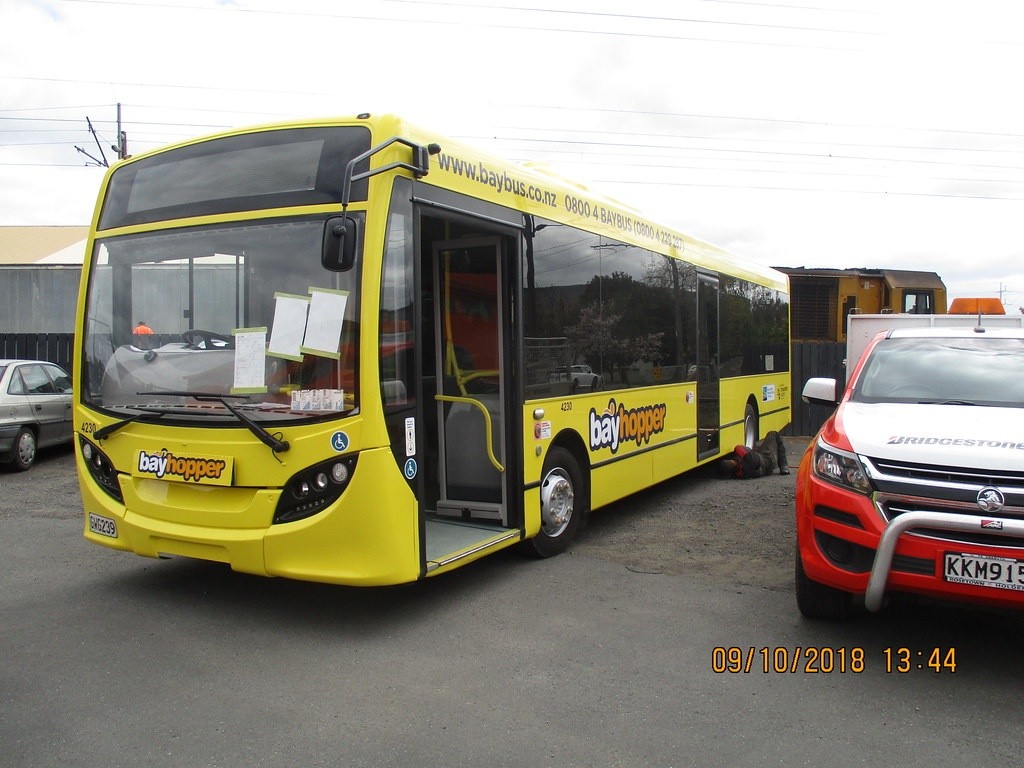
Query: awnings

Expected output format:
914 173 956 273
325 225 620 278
880 270 945 292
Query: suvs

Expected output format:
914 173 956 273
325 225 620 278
549 364 601 393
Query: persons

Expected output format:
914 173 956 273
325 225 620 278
720 430 790 479
133 321 154 348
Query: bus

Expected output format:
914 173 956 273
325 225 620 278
71 111 792 587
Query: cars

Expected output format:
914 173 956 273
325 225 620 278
795 325 1024 623
0 359 102 472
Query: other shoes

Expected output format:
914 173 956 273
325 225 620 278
780 464 791 474
